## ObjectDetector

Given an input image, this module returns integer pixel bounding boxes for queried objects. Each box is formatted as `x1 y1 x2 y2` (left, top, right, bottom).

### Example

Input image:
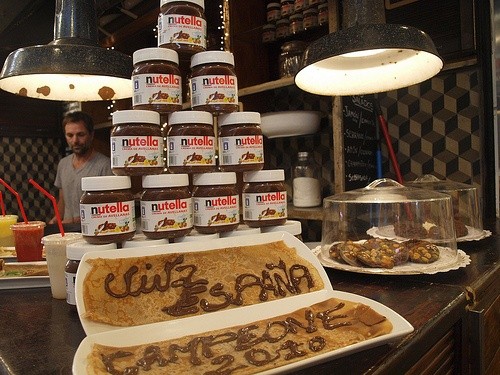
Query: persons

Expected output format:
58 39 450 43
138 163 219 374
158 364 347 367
49 111 114 225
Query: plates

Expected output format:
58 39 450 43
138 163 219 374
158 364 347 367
367 224 487 244
310 239 468 276
72 290 414 374
74 231 333 336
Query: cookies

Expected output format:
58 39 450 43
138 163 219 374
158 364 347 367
328 237 441 268
393 214 468 240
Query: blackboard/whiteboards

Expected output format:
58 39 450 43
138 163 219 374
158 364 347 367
342 92 378 191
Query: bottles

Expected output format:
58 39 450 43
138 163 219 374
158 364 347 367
279 41 306 78
292 151 321 208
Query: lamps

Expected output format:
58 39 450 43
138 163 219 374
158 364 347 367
0 0 134 103
293 0 445 99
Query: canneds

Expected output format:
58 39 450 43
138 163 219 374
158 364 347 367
261 0 328 43
64 0 302 314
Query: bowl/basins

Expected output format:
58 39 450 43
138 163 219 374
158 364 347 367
261 110 325 139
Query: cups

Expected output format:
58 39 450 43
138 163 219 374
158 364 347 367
41 232 83 299
9 221 46 263
0 215 19 247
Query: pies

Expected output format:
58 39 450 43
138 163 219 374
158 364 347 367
82 240 324 326
86 297 393 375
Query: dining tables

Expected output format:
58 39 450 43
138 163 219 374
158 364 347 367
1 234 500 375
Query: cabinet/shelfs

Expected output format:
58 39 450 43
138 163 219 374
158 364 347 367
105 0 344 244
